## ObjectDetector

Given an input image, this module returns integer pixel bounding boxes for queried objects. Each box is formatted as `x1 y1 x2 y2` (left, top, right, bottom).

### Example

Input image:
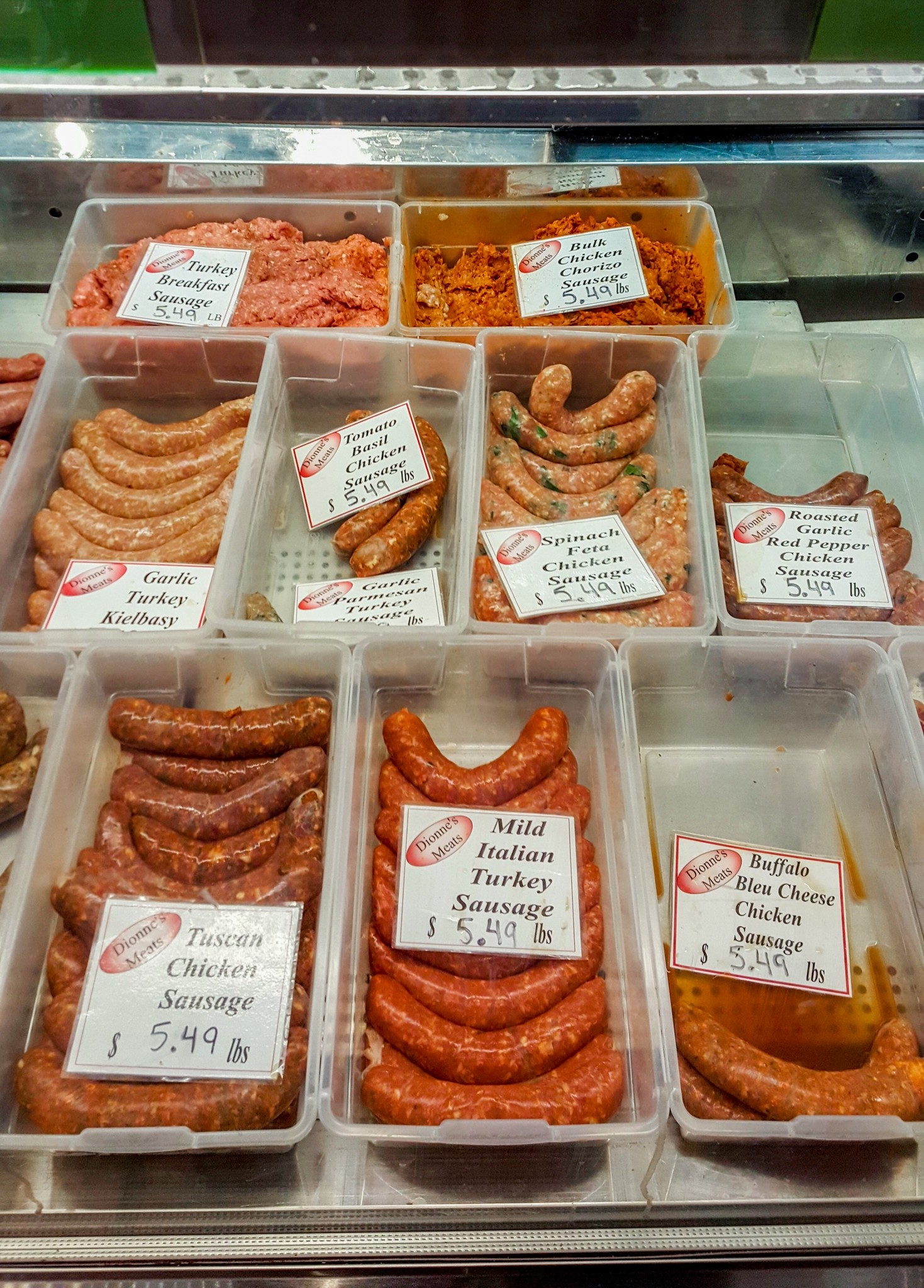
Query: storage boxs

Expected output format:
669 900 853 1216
1 164 924 1154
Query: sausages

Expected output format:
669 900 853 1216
472 364 693 627
14 696 331 1134
673 1001 924 1122
16 395 254 629
333 410 450 577
0 353 46 472
361 707 628 1125
709 453 924 625
0 691 50 907
